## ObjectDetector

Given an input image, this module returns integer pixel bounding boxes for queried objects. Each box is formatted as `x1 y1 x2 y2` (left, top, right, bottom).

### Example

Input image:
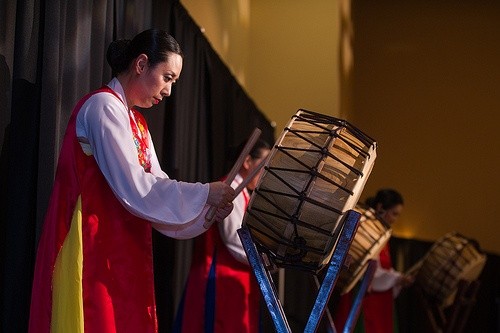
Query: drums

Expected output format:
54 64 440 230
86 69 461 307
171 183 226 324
241 108 379 274
413 230 487 309
314 201 393 296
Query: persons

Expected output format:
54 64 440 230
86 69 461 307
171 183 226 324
27 27 237 333
172 136 272 333
334 188 417 333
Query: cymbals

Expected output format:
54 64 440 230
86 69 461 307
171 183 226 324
386 228 436 244
